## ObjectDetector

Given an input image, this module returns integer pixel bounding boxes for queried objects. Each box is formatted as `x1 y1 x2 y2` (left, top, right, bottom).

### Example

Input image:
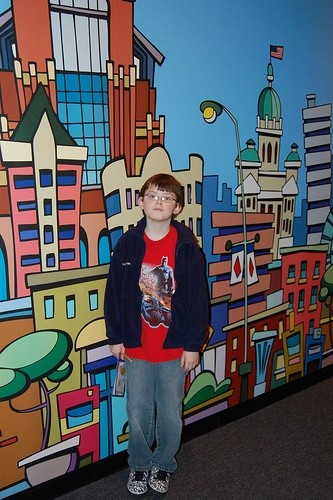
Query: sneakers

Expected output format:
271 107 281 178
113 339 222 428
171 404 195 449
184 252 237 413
148 467 171 493
127 467 149 494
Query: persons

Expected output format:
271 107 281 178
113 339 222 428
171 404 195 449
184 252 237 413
103 174 210 495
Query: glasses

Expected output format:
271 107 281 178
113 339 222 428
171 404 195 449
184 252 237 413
143 193 177 203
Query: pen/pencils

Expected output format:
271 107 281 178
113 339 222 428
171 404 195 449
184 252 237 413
120 351 133 363
